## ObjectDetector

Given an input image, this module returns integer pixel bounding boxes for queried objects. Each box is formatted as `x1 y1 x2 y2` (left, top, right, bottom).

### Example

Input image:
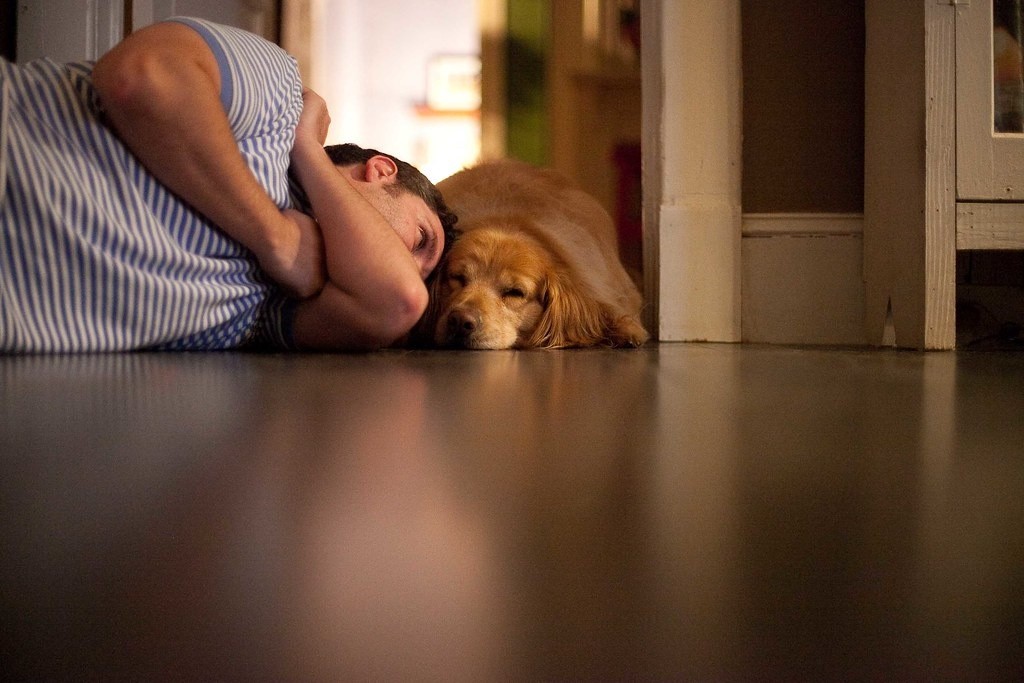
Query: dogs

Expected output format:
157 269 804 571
432 160 649 352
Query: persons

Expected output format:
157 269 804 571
0 13 465 359
